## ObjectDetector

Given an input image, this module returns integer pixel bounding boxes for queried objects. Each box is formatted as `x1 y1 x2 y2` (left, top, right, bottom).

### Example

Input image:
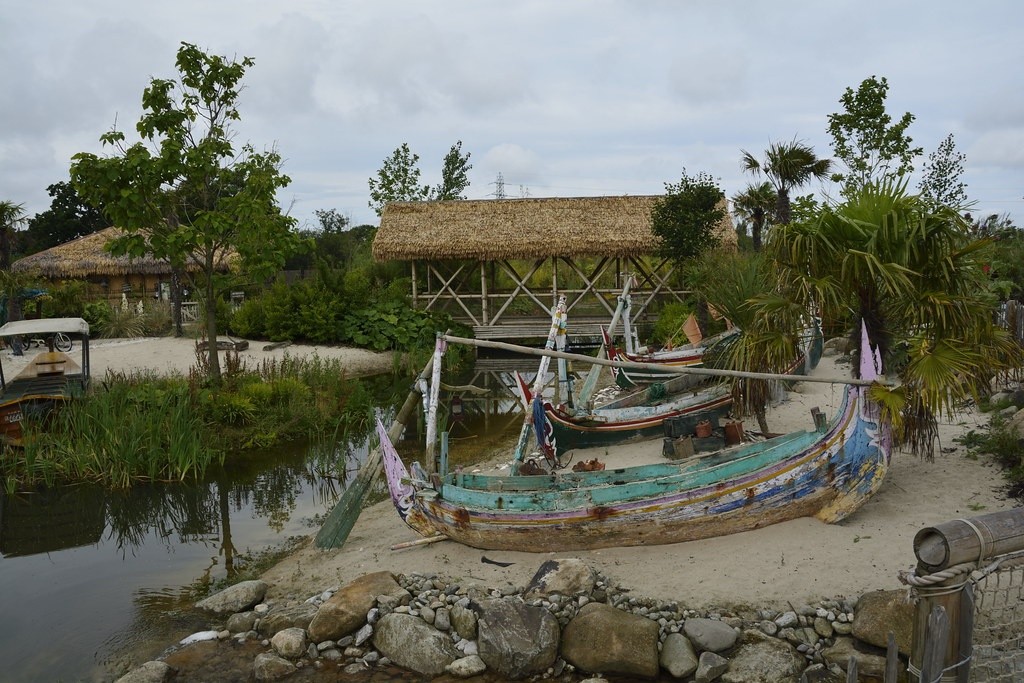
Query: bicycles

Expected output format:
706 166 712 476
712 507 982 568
8 331 72 352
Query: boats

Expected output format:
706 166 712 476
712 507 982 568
374 315 893 552
636 328 742 349
597 323 706 392
512 325 825 450
0 317 90 445
